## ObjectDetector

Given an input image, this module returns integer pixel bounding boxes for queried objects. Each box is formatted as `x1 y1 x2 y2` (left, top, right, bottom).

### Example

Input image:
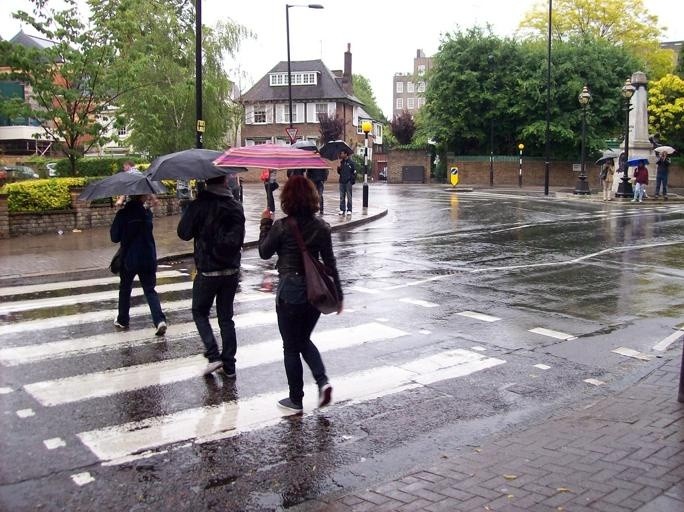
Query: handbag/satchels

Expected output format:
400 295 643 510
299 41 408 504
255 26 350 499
110 246 126 273
270 181 279 191
600 167 607 179
301 251 341 314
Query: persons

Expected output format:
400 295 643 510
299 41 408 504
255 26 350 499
336 149 357 216
109 195 168 336
631 160 648 202
653 151 672 199
115 161 160 211
601 159 616 201
306 152 329 214
176 175 246 381
259 174 345 413
287 168 305 178
260 168 279 215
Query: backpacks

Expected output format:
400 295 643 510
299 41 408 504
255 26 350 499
205 198 245 254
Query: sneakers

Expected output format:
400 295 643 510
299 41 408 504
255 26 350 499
154 321 167 335
276 398 303 414
113 318 129 328
339 210 345 215
347 211 352 215
204 359 224 376
317 382 332 408
216 366 236 378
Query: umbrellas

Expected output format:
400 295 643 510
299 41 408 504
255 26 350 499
594 154 619 166
76 170 169 202
318 139 354 167
291 140 318 151
212 142 333 171
626 156 649 167
654 145 675 155
142 148 249 180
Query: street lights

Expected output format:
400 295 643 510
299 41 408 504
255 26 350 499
360 121 375 214
573 86 592 196
484 52 496 188
284 3 323 142
516 142 525 186
614 78 637 197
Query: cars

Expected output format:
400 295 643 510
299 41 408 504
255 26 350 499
44 163 60 176
0 165 38 188
